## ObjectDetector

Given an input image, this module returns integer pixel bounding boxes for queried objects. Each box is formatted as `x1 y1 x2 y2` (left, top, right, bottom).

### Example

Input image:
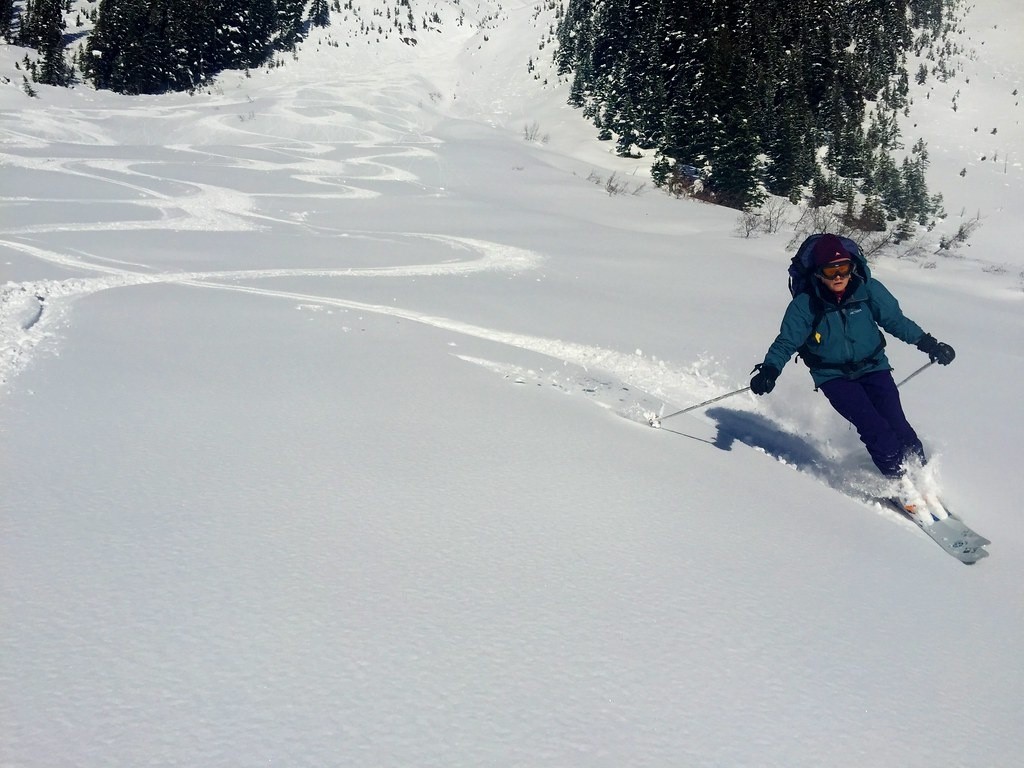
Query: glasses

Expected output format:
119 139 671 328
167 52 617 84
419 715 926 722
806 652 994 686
815 260 854 280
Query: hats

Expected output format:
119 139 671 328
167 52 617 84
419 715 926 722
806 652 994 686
813 233 851 265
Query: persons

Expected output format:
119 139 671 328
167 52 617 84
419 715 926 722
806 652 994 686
751 233 956 525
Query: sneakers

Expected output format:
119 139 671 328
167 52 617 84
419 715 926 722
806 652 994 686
916 483 949 519
890 488 933 527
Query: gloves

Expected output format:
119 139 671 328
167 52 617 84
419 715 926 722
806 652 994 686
917 333 955 366
749 363 779 395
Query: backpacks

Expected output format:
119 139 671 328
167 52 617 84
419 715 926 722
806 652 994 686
788 232 887 374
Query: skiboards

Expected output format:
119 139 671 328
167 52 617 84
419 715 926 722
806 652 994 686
891 495 991 565
